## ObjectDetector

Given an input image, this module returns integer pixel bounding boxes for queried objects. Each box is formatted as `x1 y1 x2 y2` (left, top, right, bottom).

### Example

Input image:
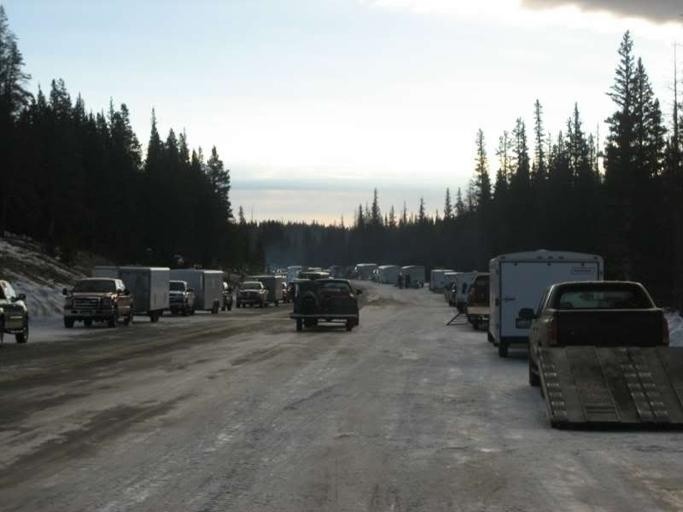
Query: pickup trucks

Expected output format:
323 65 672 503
520 279 671 389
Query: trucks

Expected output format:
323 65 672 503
402 265 426 289
377 265 400 284
489 253 605 358
117 267 171 325
172 269 225 315
249 274 284 306
430 269 492 307
356 262 376 278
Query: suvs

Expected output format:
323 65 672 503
289 278 360 332
166 279 197 318
61 277 136 330
236 281 270 310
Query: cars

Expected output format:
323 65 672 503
222 282 234 311
0 279 31 343
